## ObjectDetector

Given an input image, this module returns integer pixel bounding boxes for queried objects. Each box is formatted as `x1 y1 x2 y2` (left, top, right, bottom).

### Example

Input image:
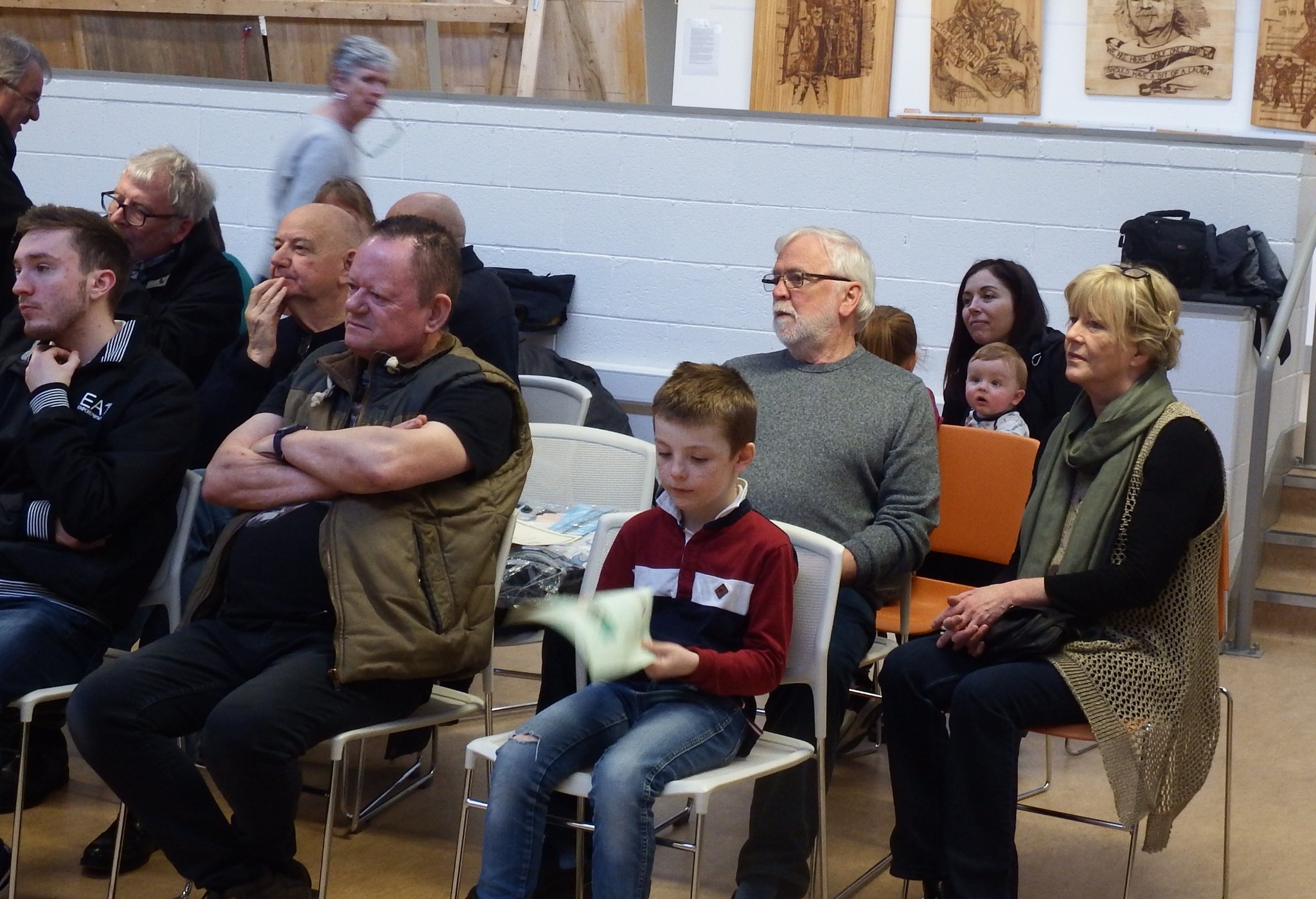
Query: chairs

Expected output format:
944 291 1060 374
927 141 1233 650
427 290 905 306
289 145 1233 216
339 373 1236 899
107 513 518 898
6 466 203 899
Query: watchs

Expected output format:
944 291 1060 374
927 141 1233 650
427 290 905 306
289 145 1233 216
273 422 309 462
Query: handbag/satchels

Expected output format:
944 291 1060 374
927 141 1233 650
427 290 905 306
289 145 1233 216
1118 209 1220 288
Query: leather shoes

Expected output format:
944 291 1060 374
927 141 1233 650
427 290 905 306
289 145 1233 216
0 754 69 815
82 813 159 879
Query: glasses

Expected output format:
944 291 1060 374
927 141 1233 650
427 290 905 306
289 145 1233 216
100 190 175 228
1110 261 1158 306
4 81 43 109
762 270 850 292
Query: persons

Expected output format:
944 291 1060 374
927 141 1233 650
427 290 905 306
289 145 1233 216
0 34 52 319
0 146 534 899
878 263 1229 899
477 362 801 899
856 260 1083 587
715 226 941 899
254 35 394 288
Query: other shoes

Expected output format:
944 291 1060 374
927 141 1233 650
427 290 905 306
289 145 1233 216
0 836 12 894
206 859 320 899
